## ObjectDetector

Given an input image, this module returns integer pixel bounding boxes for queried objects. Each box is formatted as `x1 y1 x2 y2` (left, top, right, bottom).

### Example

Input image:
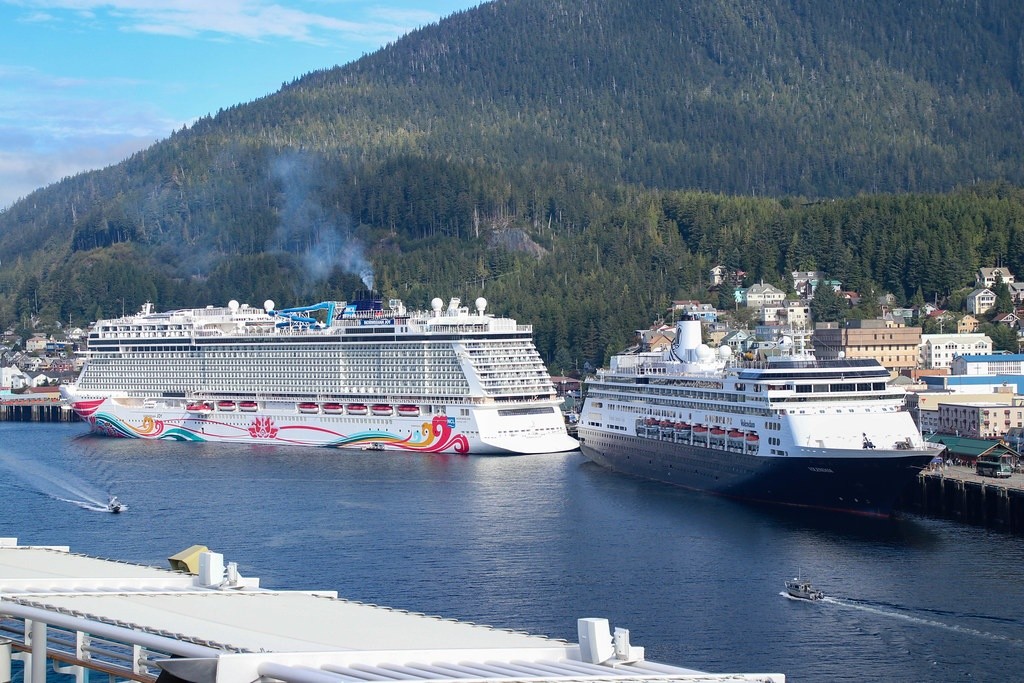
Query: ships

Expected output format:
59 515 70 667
59 298 581 457
577 320 948 493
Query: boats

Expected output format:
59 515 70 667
184 399 418 418
637 416 759 447
780 564 824 600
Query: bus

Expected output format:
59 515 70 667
974 453 1011 478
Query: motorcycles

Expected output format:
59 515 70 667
107 497 121 512
